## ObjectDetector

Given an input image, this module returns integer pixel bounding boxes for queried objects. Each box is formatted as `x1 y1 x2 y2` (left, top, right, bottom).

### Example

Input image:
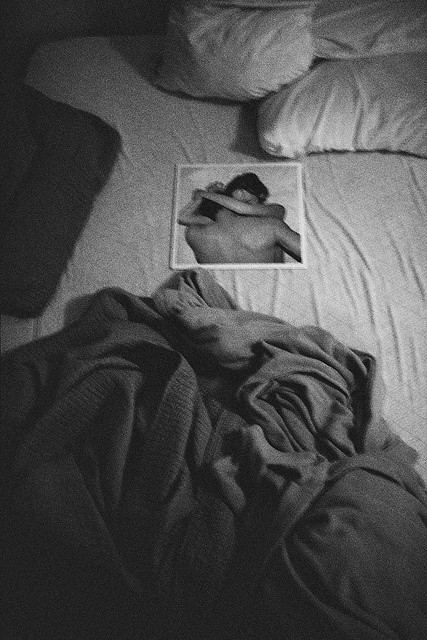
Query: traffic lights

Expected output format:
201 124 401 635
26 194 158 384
154 0 315 105
258 52 427 160
308 1 427 59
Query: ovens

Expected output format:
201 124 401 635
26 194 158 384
0 31 427 634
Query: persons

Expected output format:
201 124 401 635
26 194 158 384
185 191 302 264
178 172 286 263
